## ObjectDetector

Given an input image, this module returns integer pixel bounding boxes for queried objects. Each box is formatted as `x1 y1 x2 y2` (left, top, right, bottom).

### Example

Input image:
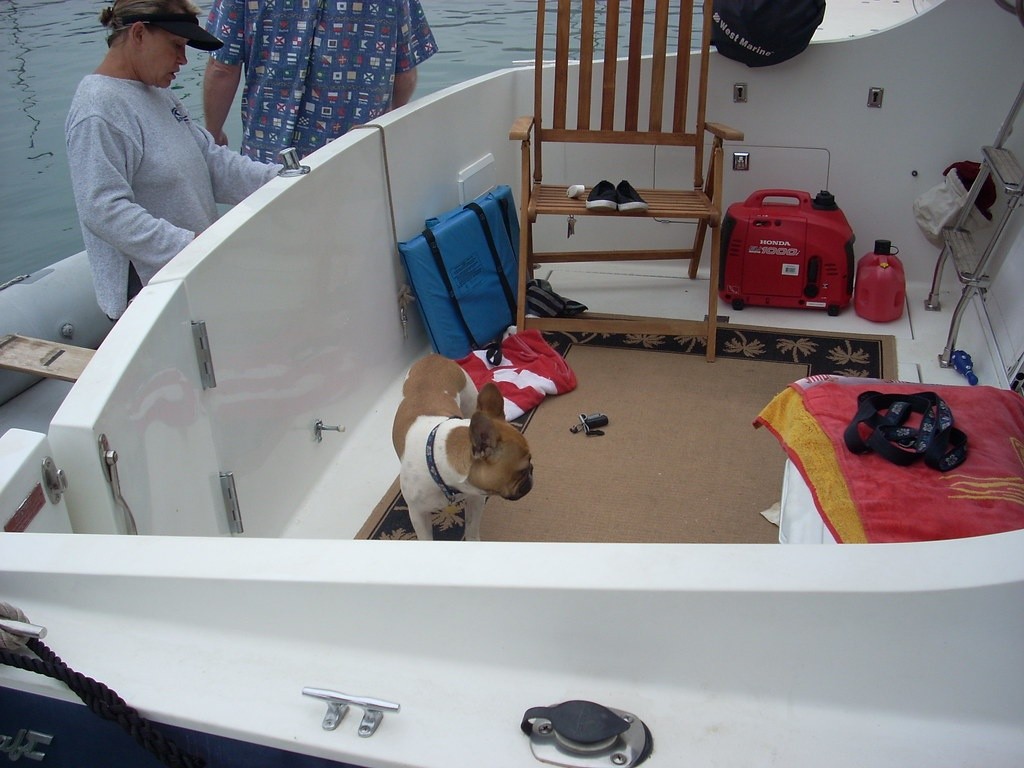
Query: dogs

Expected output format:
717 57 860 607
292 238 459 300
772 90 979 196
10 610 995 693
392 354 533 541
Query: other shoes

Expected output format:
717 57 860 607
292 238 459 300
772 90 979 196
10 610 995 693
615 180 648 211
585 180 618 211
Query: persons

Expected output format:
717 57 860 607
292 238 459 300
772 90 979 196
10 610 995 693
204 0 440 165
65 0 285 326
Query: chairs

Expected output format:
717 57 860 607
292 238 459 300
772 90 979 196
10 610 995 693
509 0 744 363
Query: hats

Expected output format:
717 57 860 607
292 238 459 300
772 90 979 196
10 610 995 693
121 11 224 51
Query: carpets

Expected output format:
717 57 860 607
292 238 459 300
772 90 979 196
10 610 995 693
353 311 898 543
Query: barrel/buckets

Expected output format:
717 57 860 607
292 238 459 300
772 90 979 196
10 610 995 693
853 239 905 323
